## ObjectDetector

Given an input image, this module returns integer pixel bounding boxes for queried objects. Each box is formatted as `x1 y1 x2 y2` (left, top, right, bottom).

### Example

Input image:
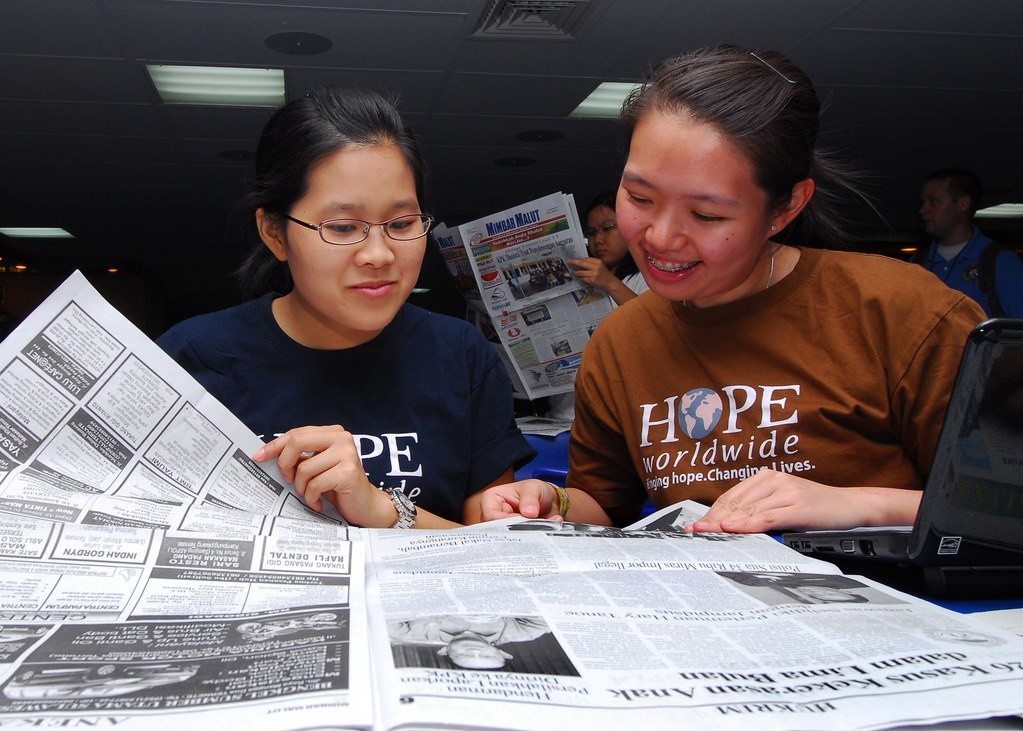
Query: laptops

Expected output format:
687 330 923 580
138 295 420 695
783 318 1023 578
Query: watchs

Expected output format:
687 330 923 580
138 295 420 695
387 488 417 529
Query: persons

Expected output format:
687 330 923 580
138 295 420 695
911 169 1023 319
155 88 539 530
545 260 567 287
716 572 868 604
577 286 604 305
387 615 551 669
479 43 988 534
545 191 649 420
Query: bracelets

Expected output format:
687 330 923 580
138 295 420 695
548 482 571 517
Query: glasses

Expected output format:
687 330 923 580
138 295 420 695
583 224 618 239
279 207 435 246
